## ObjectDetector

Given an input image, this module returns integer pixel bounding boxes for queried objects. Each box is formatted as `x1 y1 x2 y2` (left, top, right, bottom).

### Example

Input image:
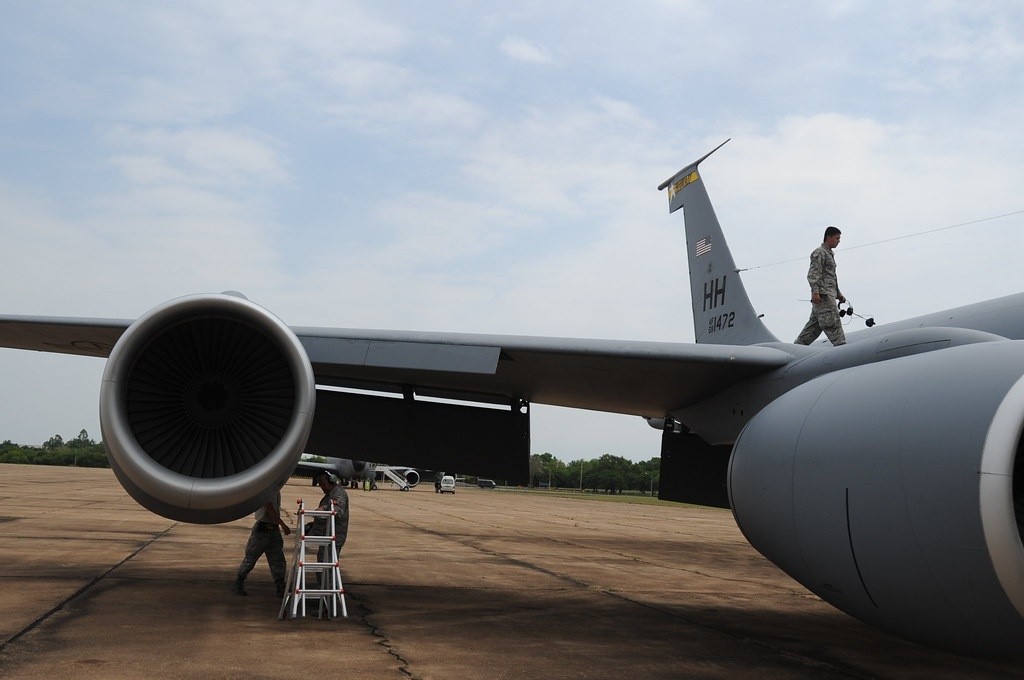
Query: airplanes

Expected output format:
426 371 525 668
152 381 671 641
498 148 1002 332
1 138 1023 665
295 456 434 492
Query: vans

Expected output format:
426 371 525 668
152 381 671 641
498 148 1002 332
478 480 496 488
440 476 455 494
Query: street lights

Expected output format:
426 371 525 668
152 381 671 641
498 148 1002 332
645 472 653 497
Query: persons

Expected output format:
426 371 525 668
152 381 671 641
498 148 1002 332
233 491 293 596
794 226 847 347
311 471 349 616
435 477 440 492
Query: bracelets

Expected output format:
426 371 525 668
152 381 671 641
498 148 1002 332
282 523 285 529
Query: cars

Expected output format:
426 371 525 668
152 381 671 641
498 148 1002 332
455 478 467 485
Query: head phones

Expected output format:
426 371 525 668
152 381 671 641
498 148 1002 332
838 300 853 317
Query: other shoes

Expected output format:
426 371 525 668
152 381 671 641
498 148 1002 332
230 581 250 597
269 583 286 601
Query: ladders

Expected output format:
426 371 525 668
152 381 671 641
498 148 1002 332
277 497 351 623
